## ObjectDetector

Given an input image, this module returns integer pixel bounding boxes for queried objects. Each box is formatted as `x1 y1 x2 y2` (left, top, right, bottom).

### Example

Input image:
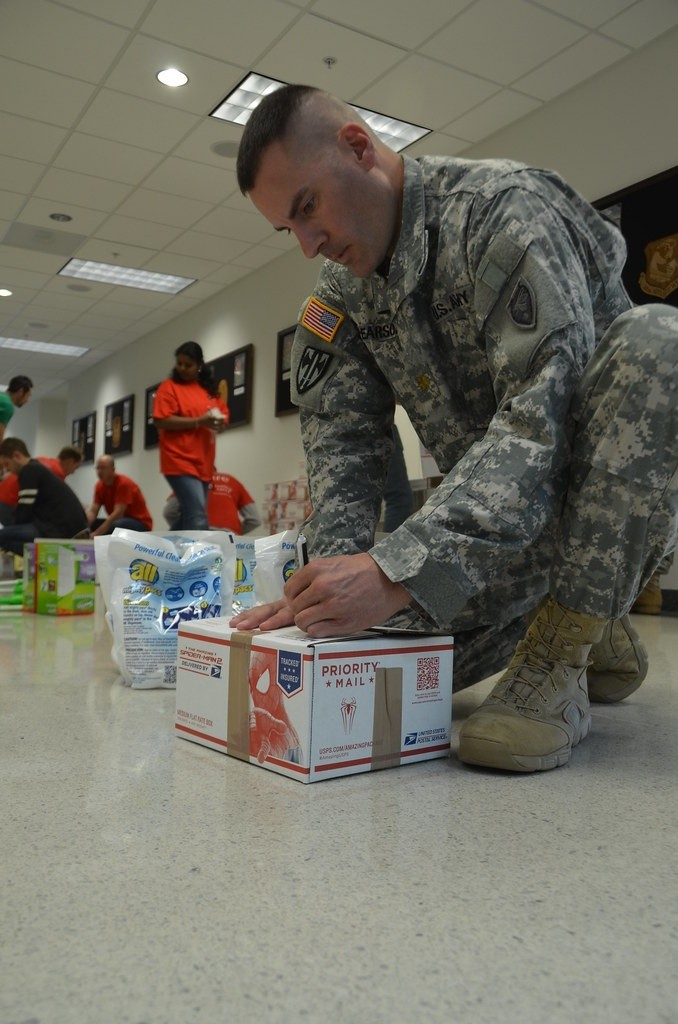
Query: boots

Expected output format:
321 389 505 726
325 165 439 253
630 574 663 614
458 595 611 772
586 612 649 703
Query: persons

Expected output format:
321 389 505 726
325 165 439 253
228 84 677 775
152 341 229 530
87 455 153 539
0 445 84 526
163 463 262 536
0 437 88 558
0 374 33 444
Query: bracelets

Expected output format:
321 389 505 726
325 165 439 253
195 416 199 430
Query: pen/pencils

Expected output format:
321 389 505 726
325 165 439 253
295 533 309 569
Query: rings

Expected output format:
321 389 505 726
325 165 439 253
215 421 216 424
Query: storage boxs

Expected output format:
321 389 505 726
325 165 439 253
262 462 312 537
176 615 453 785
21 539 95 616
0 554 16 580
